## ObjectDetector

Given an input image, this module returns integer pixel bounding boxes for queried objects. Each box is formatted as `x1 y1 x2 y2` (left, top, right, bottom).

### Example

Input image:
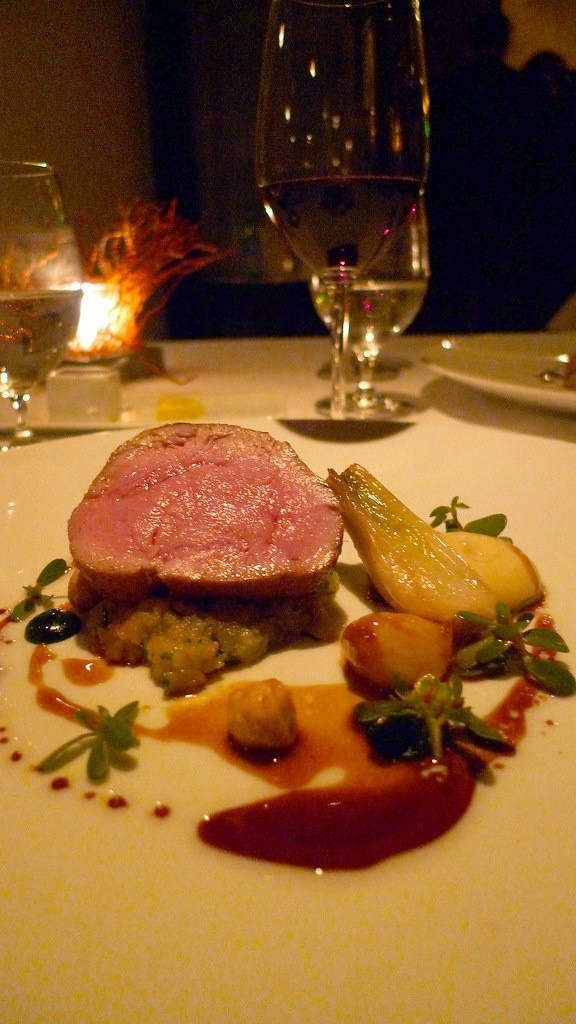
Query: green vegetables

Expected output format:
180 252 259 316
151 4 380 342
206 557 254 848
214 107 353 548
10 496 576 782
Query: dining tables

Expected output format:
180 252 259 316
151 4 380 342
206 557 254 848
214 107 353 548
0 334 575 1024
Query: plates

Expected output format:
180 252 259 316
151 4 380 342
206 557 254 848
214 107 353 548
0 417 576 1024
419 332 576 413
29 412 150 431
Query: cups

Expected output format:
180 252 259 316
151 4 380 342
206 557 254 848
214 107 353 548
47 364 123 428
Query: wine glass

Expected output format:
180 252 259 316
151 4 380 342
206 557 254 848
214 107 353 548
0 160 83 452
255 2 429 420
310 190 420 419
